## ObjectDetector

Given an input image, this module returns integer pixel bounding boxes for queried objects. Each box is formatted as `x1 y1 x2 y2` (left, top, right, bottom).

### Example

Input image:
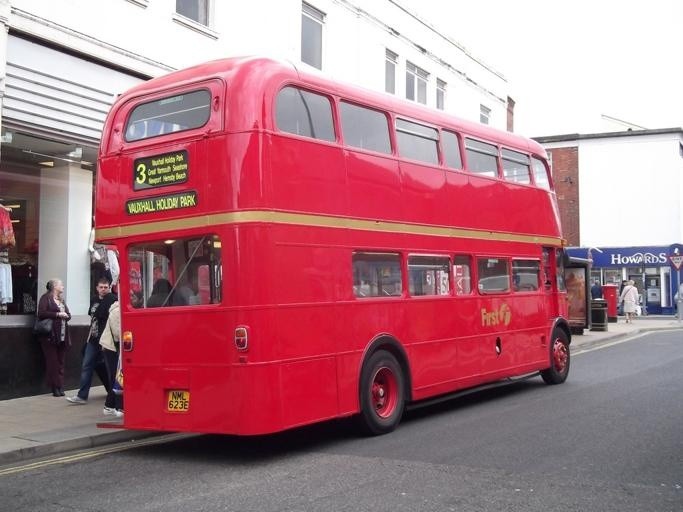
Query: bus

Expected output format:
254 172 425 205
89 55 573 439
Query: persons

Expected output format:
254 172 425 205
0 198 17 315
619 280 628 317
619 280 640 324
67 266 175 404
591 280 602 298
88 215 120 297
38 279 72 397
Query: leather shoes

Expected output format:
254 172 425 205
53 387 65 396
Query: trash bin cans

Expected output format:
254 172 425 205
589 298 608 331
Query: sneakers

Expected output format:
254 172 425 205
66 396 87 404
103 405 124 418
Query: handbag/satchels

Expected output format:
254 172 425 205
32 318 53 336
